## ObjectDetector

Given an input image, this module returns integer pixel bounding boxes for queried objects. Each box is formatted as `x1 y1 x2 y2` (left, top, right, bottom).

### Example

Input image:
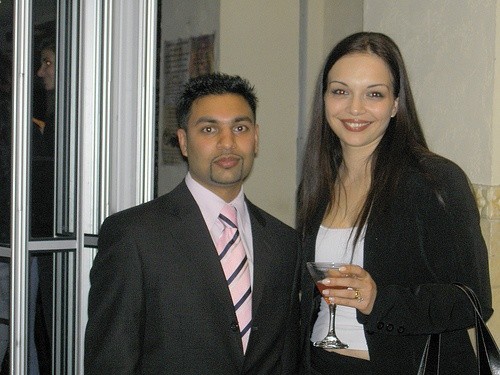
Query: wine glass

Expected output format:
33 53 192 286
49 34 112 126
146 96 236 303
305 262 357 348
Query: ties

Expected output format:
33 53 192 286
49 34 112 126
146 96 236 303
216 204 252 355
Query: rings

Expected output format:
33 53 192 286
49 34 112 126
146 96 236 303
355 289 358 299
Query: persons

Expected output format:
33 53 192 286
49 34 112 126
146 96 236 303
82 71 302 375
296 31 495 375
32 39 56 375
